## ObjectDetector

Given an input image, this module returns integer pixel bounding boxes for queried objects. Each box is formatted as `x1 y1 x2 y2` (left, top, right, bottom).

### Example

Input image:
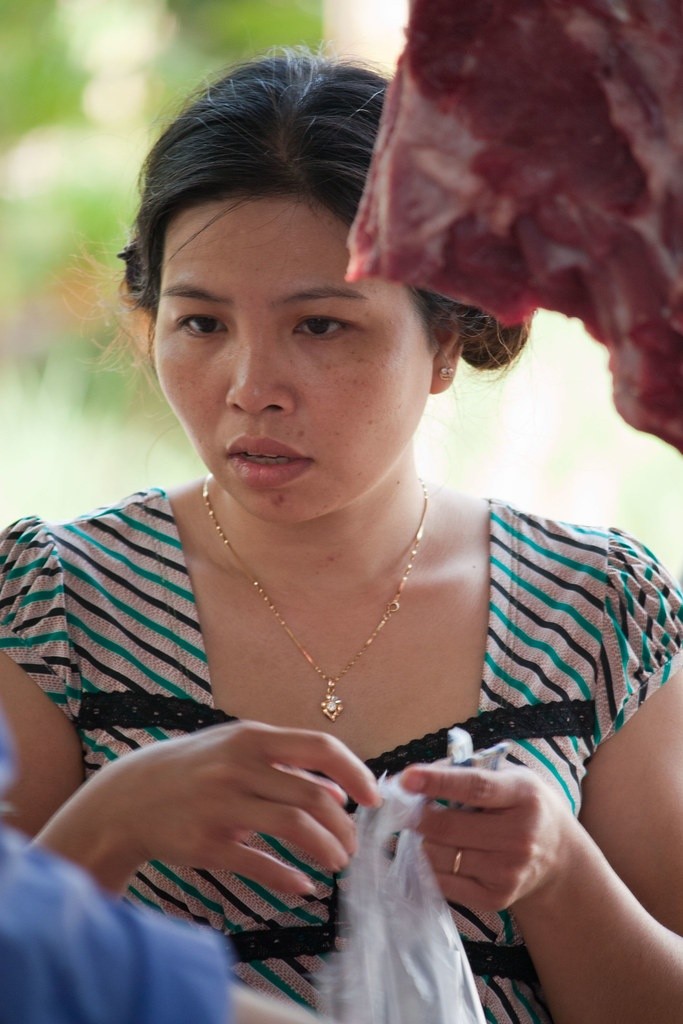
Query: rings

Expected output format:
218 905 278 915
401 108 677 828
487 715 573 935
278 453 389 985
453 850 463 876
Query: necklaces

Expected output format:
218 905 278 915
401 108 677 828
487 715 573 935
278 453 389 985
203 474 428 720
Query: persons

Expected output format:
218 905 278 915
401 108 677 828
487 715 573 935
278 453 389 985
1 53 683 1024
1 714 325 1024
